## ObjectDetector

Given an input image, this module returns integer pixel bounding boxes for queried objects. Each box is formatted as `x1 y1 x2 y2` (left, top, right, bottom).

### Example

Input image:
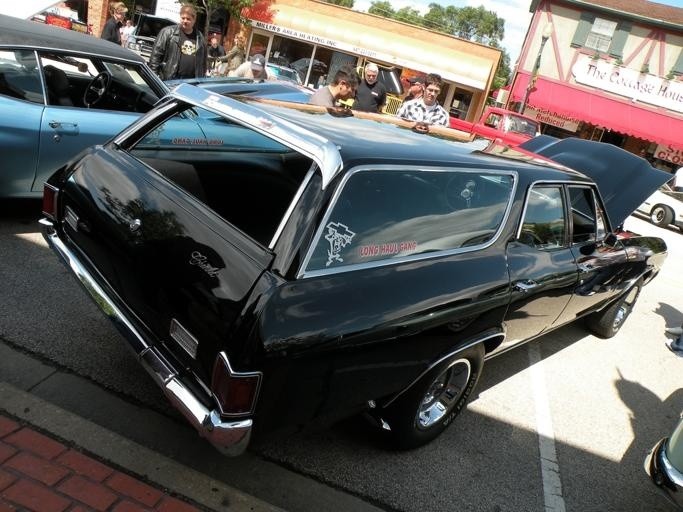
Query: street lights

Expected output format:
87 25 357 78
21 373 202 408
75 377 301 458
518 23 554 113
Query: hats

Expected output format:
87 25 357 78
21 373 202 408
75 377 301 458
406 76 426 84
251 54 266 72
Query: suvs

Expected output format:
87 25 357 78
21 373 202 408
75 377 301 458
38 82 683 459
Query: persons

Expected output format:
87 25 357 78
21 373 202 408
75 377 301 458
234 54 268 81
208 39 226 59
309 64 361 109
217 34 249 76
101 2 129 45
401 77 426 104
351 61 387 113
396 73 450 128
121 22 136 36
147 5 208 80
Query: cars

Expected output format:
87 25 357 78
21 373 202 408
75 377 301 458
632 184 683 231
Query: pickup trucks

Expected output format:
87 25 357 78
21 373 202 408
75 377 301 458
448 107 541 150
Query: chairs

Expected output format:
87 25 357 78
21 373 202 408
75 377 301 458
42 62 75 108
